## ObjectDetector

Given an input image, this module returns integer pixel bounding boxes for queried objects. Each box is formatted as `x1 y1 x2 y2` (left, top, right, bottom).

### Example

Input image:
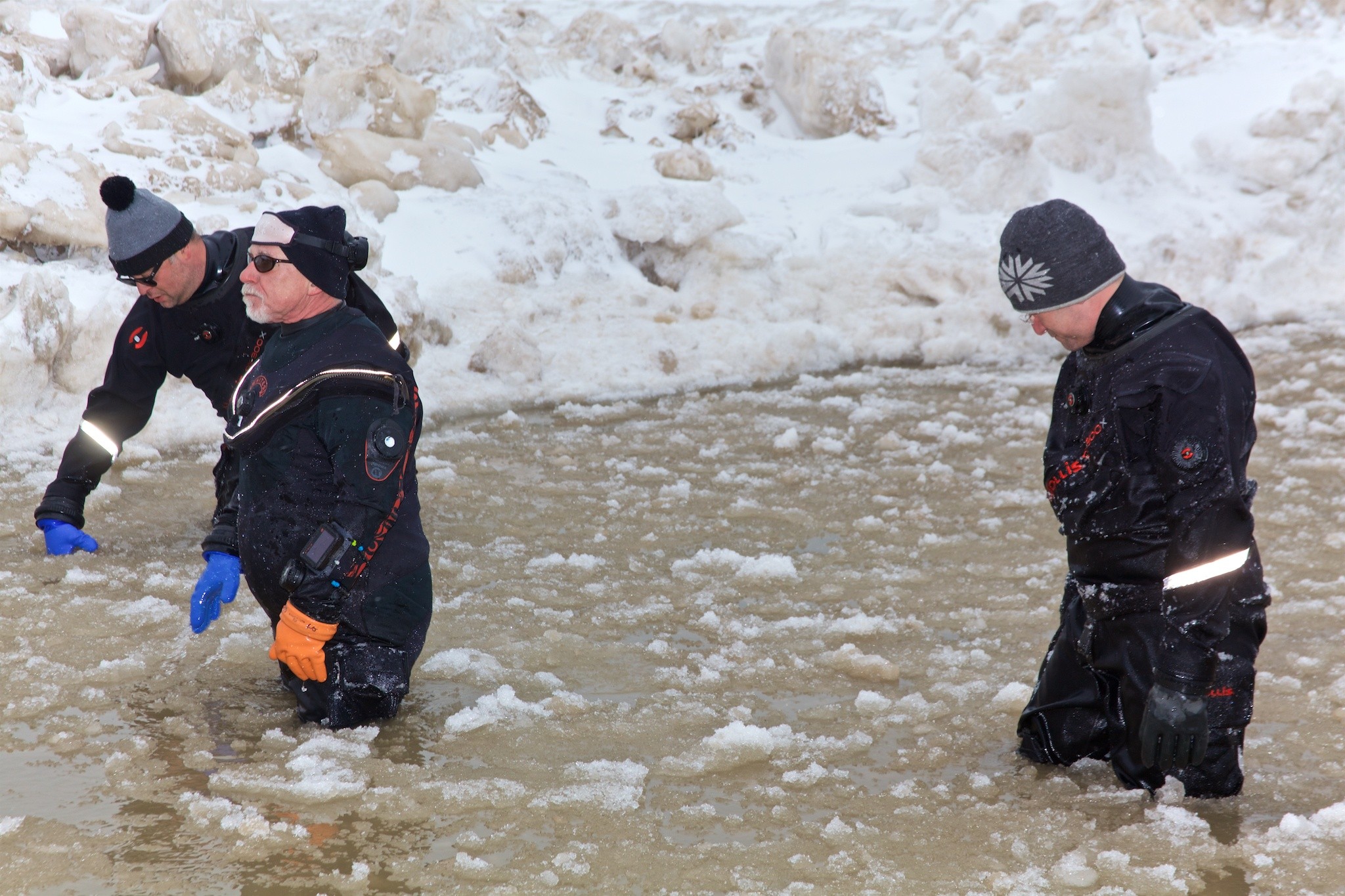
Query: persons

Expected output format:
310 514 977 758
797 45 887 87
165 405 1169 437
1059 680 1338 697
190 206 432 732
998 198 1272 802
33 175 411 555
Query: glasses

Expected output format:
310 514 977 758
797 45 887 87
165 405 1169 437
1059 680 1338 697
1021 311 1035 325
116 261 164 287
247 252 293 273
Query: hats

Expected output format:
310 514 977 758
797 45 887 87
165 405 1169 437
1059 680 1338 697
99 175 193 275
998 199 1127 313
273 205 350 300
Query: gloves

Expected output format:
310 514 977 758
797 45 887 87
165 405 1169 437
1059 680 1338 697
268 600 338 681
1139 651 1219 770
36 518 98 556
190 551 240 634
